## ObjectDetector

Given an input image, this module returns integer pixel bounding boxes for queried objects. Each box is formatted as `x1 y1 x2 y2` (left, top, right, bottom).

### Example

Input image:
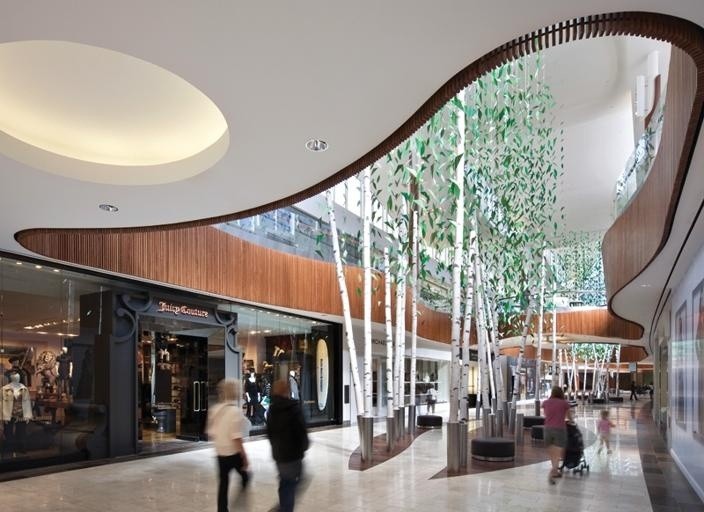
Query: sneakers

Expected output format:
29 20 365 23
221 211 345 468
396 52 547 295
598 446 613 455
547 469 561 486
242 471 253 489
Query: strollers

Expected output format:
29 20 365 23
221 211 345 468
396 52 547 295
558 400 590 477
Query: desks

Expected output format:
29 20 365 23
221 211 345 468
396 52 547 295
35 398 72 423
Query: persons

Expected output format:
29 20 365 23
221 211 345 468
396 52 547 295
426 384 439 414
288 371 301 401
245 371 261 418
265 378 310 511
629 380 639 400
648 381 654 398
1 348 76 439
542 388 576 488
202 378 254 512
595 409 616 457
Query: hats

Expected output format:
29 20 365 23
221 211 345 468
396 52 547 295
4 366 24 377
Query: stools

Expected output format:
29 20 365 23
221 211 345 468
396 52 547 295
416 414 442 429
530 424 544 442
521 415 544 427
470 436 515 462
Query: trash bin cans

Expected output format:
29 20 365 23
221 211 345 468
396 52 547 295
469 394 478 409
